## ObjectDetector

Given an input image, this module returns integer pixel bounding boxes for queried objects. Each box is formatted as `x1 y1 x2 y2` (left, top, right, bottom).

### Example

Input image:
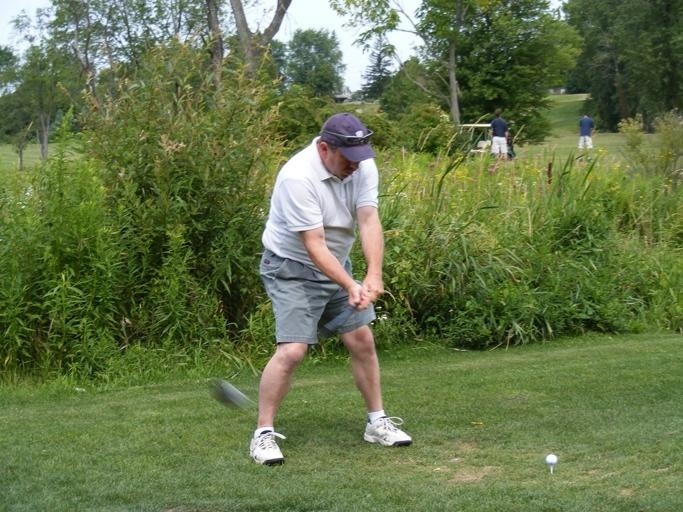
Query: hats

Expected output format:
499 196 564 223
318 111 378 164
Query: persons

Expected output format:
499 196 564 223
247 112 414 463
488 108 509 161
574 111 596 152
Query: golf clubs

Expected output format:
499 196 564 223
207 303 357 409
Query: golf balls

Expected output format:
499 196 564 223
545 454 557 465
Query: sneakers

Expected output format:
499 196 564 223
248 429 287 467
363 414 414 448
